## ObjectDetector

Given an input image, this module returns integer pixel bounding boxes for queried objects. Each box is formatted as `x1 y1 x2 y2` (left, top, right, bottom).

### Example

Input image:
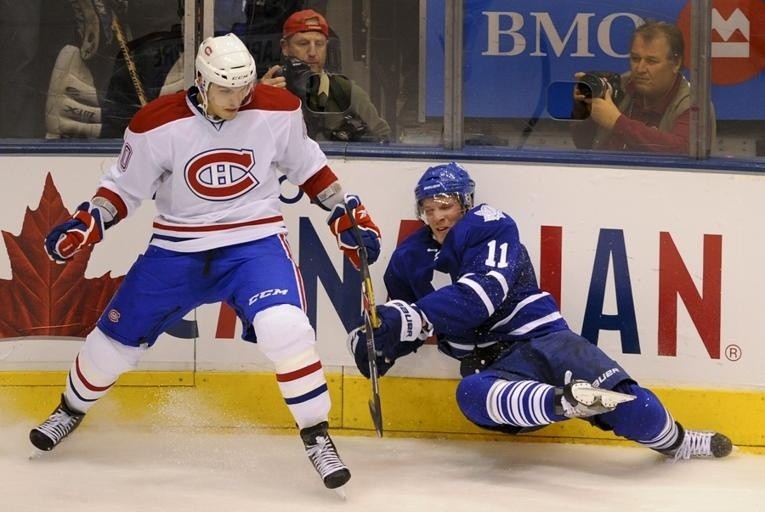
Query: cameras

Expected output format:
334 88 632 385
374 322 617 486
577 70 624 107
270 56 321 95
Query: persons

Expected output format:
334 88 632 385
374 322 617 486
93 1 213 139
258 9 393 144
350 158 733 462
28 30 381 490
570 20 718 157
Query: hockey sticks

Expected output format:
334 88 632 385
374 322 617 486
363 309 383 438
345 203 382 327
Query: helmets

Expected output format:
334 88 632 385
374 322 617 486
416 163 476 200
195 33 256 110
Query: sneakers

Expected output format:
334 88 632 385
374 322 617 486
28 396 85 450
655 422 733 459
298 421 350 489
553 379 615 419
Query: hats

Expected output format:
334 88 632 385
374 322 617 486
282 9 329 39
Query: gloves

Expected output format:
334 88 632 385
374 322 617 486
371 298 434 360
328 194 382 272
345 324 398 380
43 200 106 266
